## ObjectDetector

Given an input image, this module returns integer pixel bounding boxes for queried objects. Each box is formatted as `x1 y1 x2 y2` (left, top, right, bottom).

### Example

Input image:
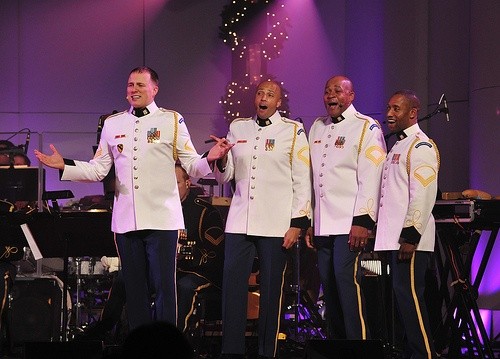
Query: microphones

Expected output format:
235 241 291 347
443 95 449 122
339 100 351 107
24 130 31 155
382 120 388 124
126 96 129 99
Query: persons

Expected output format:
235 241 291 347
308 76 387 340
373 88 441 359
210 80 312 359
34 65 235 332
86 161 223 336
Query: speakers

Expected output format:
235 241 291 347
3 279 62 347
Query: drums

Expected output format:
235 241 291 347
74 258 105 275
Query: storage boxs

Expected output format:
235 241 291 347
432 199 500 223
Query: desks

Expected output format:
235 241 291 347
434 223 500 359
